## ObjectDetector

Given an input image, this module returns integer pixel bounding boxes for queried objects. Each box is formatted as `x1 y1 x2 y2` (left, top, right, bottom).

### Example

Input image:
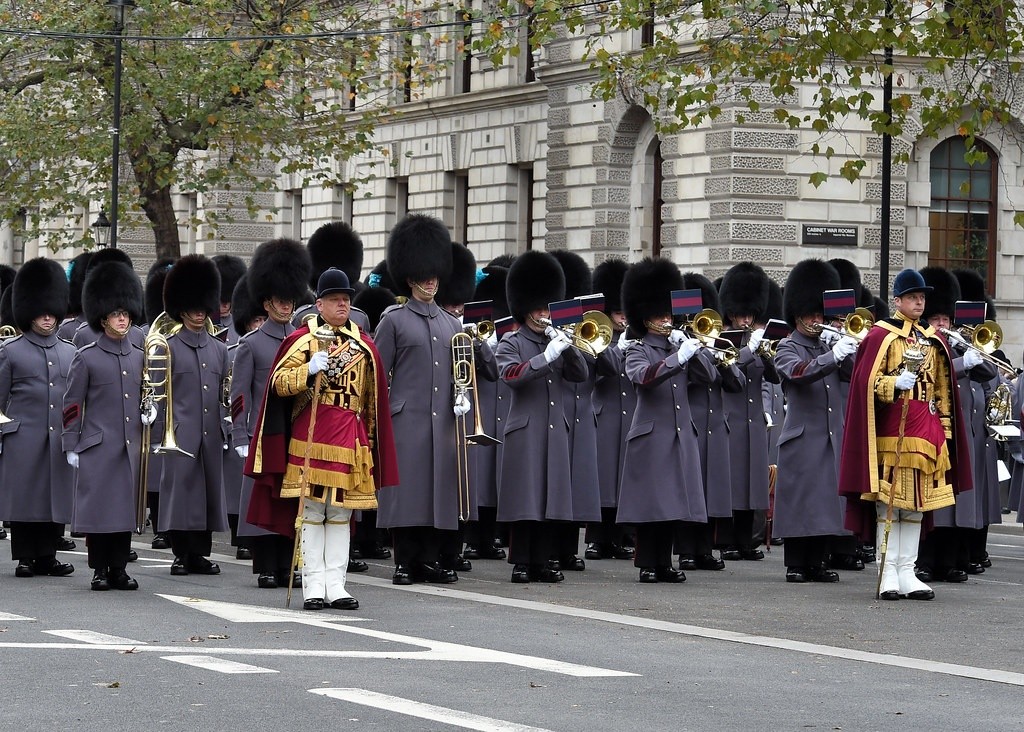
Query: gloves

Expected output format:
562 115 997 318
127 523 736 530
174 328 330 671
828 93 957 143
668 329 688 348
765 412 773 428
706 341 719 356
748 329 765 353
141 405 157 425
234 444 249 458
66 450 80 468
714 351 736 366
309 352 330 375
895 364 918 390
817 324 842 346
677 338 703 366
964 343 983 370
544 336 572 363
946 330 966 349
834 336 859 361
453 395 471 415
783 404 789 412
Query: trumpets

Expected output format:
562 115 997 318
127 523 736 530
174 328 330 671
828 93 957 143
221 361 233 424
745 323 781 361
621 320 629 331
213 325 220 334
454 309 494 342
394 296 410 304
715 347 740 367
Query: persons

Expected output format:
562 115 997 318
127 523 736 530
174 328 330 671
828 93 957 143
772 259 854 583
372 214 473 585
60 261 160 592
839 269 974 601
495 251 589 583
2 249 1024 583
242 266 401 609
616 258 708 584
229 238 311 588
290 220 370 573
1 257 78 579
152 254 230 576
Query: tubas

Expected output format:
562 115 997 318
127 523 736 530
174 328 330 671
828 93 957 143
148 311 214 340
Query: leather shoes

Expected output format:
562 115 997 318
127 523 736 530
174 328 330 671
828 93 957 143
0 505 1022 611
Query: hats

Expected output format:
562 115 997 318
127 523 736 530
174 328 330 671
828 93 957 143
1 212 997 334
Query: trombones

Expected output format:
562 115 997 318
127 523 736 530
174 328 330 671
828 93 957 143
662 308 737 355
300 313 319 325
812 306 876 343
134 332 196 536
451 331 503 523
538 310 614 360
0 324 17 342
0 409 14 424
937 319 1018 377
